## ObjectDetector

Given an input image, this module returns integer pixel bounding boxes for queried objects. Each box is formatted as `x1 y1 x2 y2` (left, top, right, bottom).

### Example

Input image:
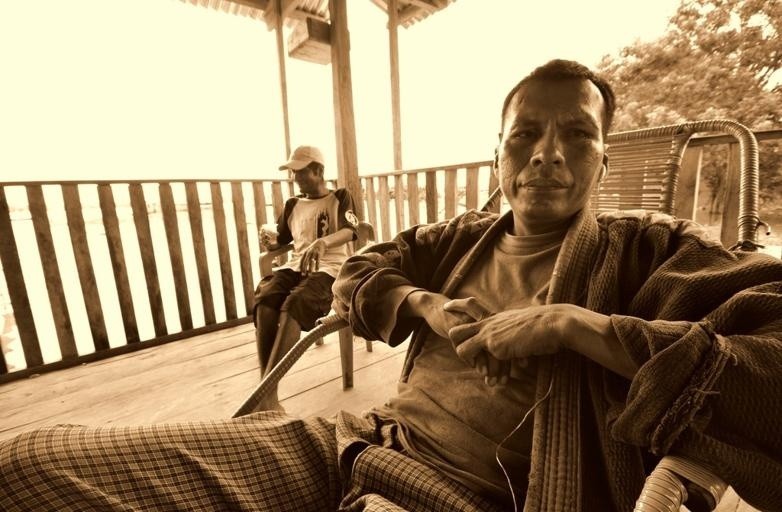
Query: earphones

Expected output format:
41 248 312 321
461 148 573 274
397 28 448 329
598 164 607 184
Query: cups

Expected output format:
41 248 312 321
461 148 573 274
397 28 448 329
262 223 279 245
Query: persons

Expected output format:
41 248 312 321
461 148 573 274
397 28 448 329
251 143 361 415
1 58 782 511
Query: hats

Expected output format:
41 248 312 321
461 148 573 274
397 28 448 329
279 146 325 170
